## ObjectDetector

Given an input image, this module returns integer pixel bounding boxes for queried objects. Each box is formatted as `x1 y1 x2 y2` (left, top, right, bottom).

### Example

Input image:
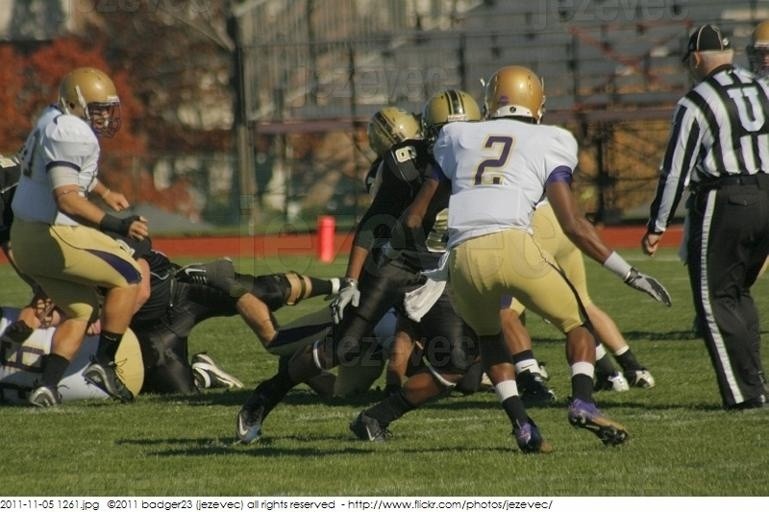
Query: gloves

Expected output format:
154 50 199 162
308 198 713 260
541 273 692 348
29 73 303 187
621 267 672 308
328 277 360 324
372 238 402 270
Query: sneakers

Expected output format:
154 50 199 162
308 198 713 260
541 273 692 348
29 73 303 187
323 277 351 301
515 367 655 452
236 383 278 444
23 380 59 408
349 410 392 445
189 350 243 391
80 355 134 401
727 394 766 409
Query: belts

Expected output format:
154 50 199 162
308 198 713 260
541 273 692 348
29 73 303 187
691 173 762 191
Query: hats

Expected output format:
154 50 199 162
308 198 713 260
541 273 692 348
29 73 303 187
680 23 733 61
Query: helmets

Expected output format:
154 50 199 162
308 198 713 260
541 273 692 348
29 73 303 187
367 107 422 158
746 21 769 85
478 65 545 124
56 69 123 138
423 88 478 151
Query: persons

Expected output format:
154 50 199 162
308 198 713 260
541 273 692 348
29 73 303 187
640 21 769 411
752 21 769 74
0 106 671 407
400 63 676 455
228 88 481 442
9 67 155 408
